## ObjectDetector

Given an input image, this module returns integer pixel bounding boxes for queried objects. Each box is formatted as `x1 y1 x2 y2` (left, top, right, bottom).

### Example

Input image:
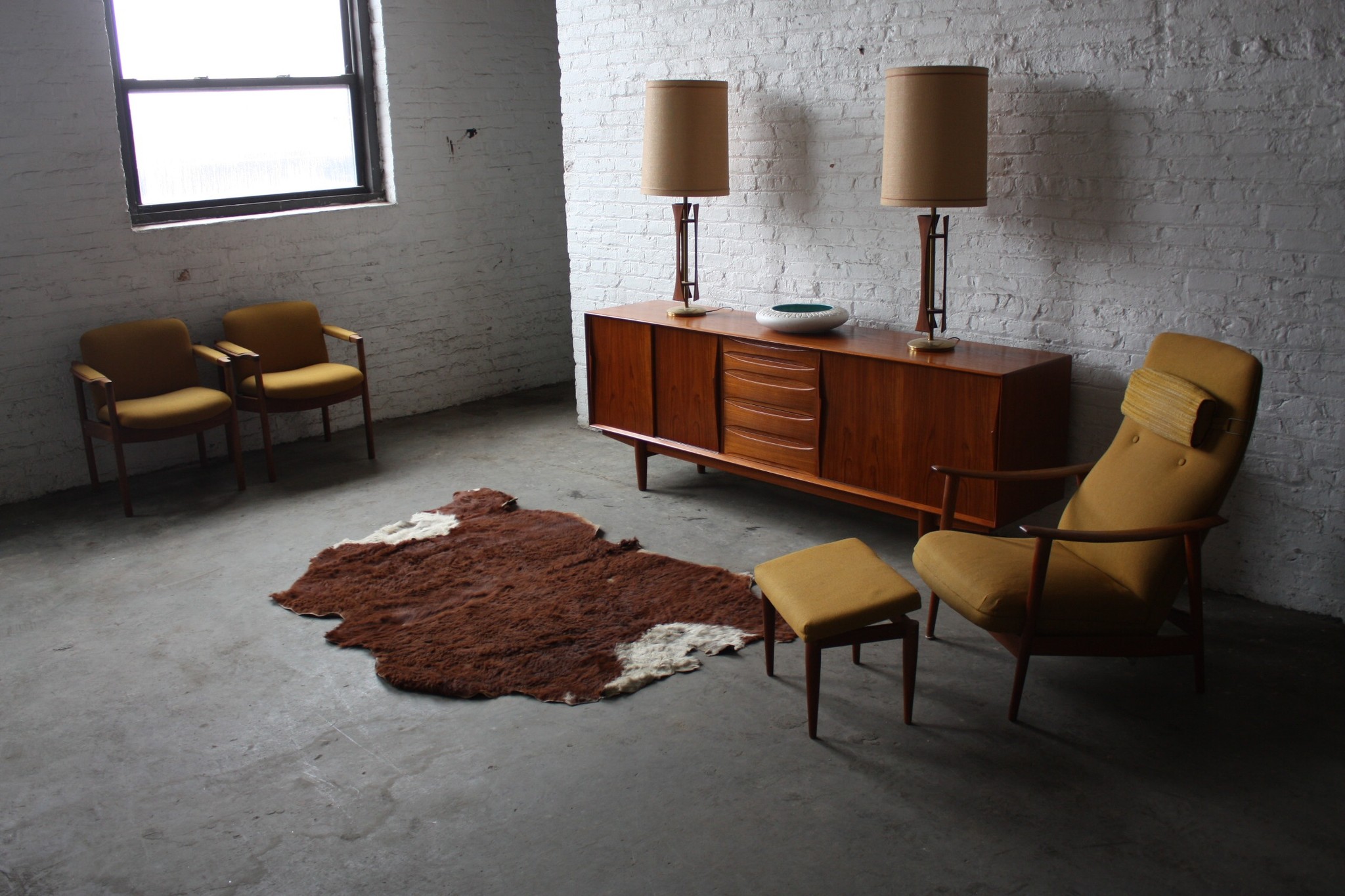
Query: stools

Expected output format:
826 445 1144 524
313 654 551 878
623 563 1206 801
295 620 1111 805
755 536 922 738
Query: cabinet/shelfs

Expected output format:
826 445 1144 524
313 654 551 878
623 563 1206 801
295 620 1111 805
582 299 1072 539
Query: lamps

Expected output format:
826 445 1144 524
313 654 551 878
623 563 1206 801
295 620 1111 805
641 79 730 316
879 65 988 351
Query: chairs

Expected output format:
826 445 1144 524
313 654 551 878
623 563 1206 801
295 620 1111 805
914 332 1265 723
70 317 246 517
215 301 377 480
